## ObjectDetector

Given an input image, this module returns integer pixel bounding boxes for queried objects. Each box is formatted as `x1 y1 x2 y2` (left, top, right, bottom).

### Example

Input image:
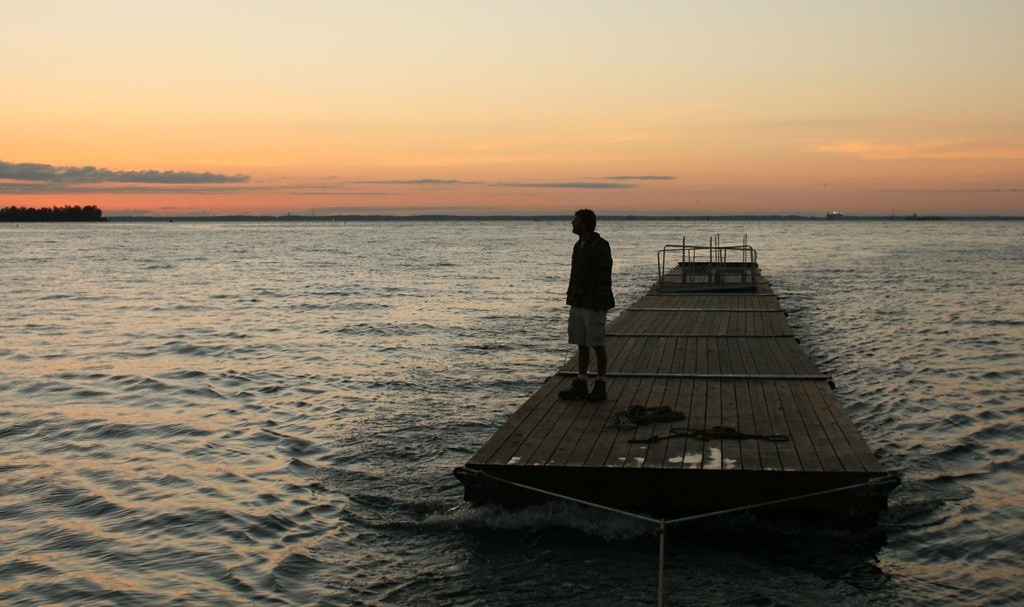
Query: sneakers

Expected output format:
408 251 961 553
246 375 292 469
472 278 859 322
583 379 607 402
557 378 588 399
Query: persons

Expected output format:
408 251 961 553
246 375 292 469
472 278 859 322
558 208 616 403
